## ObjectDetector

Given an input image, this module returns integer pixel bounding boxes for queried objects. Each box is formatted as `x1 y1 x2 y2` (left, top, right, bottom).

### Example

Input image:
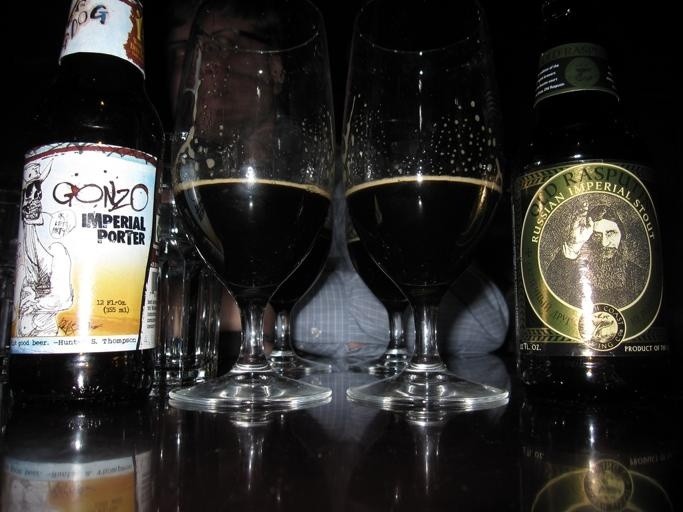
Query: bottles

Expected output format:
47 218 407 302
519 402 674 512
9 0 166 404
0 405 160 512
510 0 669 400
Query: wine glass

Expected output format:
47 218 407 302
342 0 504 404
267 202 334 373
169 396 333 512
344 204 413 371
169 0 335 403
346 396 508 512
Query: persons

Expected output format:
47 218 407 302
547 200 648 309
153 17 509 357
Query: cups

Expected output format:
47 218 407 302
0 356 19 511
0 189 21 355
152 38 222 388
